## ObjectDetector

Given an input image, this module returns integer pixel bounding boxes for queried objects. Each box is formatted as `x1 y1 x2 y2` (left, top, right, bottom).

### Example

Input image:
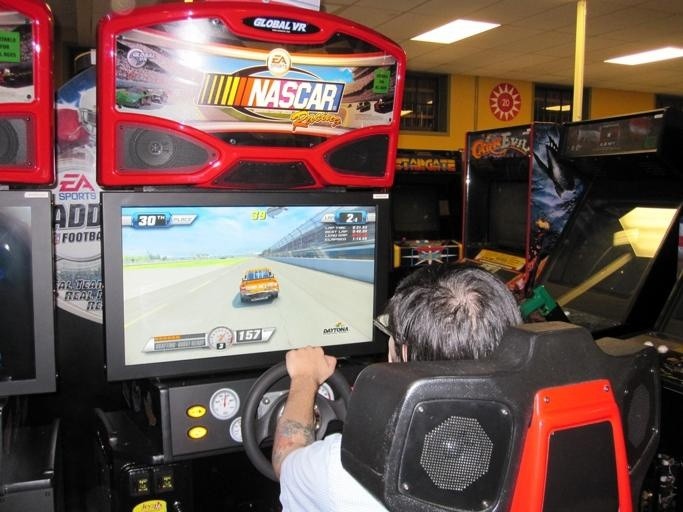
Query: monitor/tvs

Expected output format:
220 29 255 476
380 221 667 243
464 155 530 257
99 192 393 381
0 191 56 398
391 181 451 232
547 196 679 297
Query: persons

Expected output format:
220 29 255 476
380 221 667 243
273 260 524 512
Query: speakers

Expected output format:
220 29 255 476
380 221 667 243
0 117 27 166
115 124 219 170
322 136 387 176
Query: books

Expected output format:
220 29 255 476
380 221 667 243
456 247 529 294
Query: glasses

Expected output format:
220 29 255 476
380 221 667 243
371 312 406 342
